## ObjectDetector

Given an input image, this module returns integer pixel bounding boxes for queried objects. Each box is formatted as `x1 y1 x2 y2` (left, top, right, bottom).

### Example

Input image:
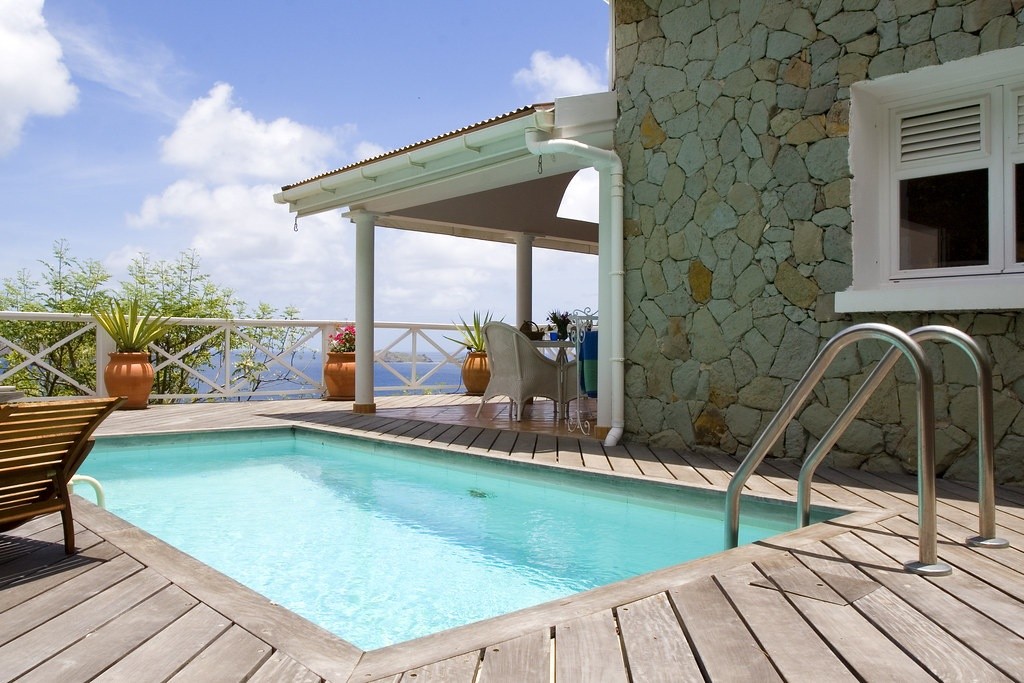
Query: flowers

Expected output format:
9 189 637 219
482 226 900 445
328 325 356 351
546 311 571 322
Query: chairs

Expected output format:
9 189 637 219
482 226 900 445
475 321 563 422
0 396 127 554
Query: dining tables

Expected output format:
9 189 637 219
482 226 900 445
530 339 576 365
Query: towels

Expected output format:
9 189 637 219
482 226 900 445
577 330 597 399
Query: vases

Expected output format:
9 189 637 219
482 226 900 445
324 352 355 401
557 322 567 340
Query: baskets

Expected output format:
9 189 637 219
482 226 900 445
520 320 545 340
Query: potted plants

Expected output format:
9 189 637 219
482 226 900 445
89 299 183 410
443 310 505 395
